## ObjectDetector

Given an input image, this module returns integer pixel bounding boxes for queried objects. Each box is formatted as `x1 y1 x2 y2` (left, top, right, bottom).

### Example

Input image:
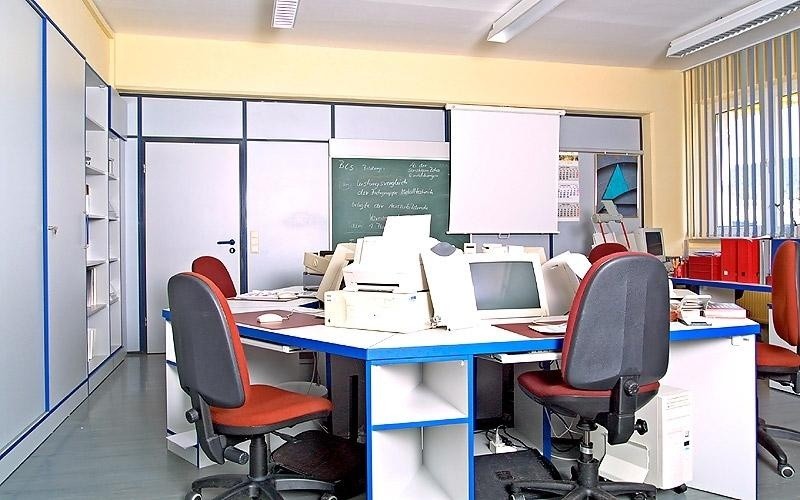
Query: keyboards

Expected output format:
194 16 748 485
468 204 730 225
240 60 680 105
528 325 566 333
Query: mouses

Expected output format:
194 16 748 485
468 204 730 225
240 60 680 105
278 292 298 299
257 311 284 325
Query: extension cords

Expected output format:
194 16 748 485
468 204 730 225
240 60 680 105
490 441 517 453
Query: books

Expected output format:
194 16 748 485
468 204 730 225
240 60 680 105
86 267 96 307
88 328 96 362
705 302 746 319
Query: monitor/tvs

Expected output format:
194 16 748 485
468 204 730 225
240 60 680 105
543 250 580 315
314 243 355 302
634 226 665 260
459 254 549 319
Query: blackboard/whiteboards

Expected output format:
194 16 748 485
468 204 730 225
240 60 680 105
329 156 472 252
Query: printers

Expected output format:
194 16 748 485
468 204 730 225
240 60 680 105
339 237 454 292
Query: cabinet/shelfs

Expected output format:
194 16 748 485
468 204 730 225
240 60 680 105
85 62 127 399
1 1 86 487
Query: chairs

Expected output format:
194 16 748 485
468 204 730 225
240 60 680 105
168 272 338 500
508 252 671 500
755 240 800 479
192 255 237 299
588 243 628 265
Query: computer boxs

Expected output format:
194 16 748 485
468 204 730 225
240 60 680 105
591 384 695 491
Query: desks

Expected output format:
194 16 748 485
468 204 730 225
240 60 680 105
162 287 760 500
669 276 772 303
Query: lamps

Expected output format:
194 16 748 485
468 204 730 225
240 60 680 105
486 0 566 44
271 0 300 30
666 0 800 58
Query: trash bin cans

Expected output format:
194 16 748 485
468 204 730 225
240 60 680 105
270 382 329 437
